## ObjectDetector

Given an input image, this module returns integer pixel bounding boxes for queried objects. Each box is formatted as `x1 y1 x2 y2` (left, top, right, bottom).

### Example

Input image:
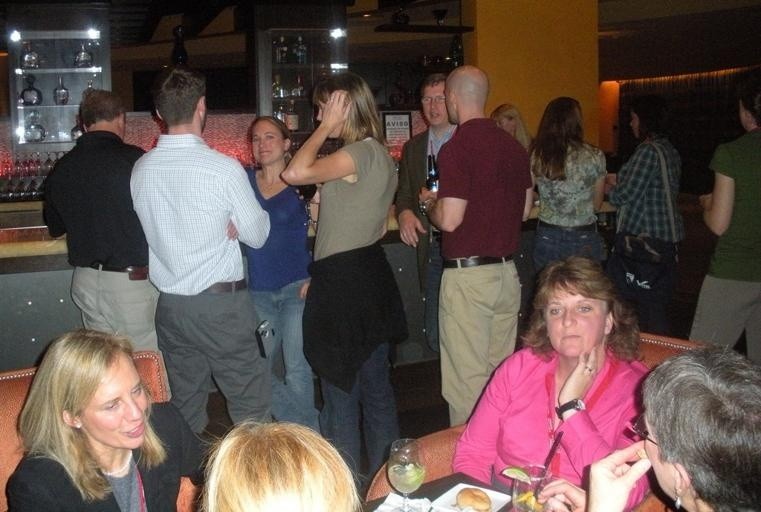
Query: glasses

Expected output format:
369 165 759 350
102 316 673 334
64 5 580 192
420 96 446 103
633 414 658 445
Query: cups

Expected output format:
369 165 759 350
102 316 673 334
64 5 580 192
514 463 555 512
606 173 617 184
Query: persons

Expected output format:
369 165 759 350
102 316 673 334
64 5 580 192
527 96 607 281
127 66 289 454
196 419 362 512
37 87 161 356
537 344 761 512
230 111 321 429
394 63 534 428
602 95 686 336
687 82 761 365
280 67 415 488
3 330 215 511
456 254 655 512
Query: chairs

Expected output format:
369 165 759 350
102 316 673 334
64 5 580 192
640 333 698 377
0 350 181 509
369 336 707 509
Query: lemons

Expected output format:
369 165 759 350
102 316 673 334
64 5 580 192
503 467 530 483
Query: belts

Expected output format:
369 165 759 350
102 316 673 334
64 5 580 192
538 220 595 232
93 261 145 272
441 257 512 267
206 279 249 295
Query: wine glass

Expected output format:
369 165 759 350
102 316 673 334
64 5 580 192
388 438 427 511
0 149 72 175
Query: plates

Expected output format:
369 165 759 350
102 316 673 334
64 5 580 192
429 482 513 512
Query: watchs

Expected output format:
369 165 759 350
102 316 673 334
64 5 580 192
555 399 585 419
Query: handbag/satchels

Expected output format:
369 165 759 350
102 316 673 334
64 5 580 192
608 231 677 297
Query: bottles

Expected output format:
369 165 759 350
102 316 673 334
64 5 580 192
51 73 70 106
451 39 464 69
19 76 41 107
0 172 48 199
23 111 46 143
423 153 442 194
82 77 96 99
172 25 188 66
68 113 87 140
20 39 42 70
272 34 336 133
398 7 410 25
75 42 94 67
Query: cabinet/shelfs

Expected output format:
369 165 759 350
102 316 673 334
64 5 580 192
12 26 102 149
270 24 347 139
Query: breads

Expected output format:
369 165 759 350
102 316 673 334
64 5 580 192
458 488 491 512
